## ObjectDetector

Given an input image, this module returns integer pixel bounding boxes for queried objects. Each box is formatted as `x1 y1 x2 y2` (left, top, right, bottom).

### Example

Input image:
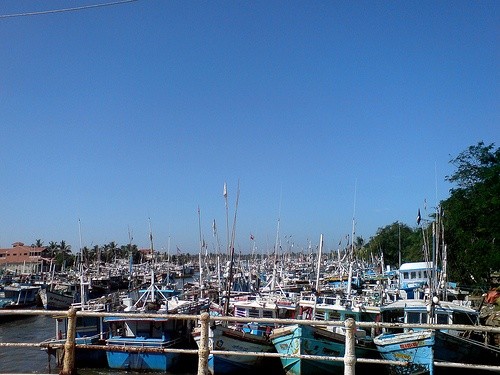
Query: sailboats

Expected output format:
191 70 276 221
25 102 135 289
0 163 480 374
